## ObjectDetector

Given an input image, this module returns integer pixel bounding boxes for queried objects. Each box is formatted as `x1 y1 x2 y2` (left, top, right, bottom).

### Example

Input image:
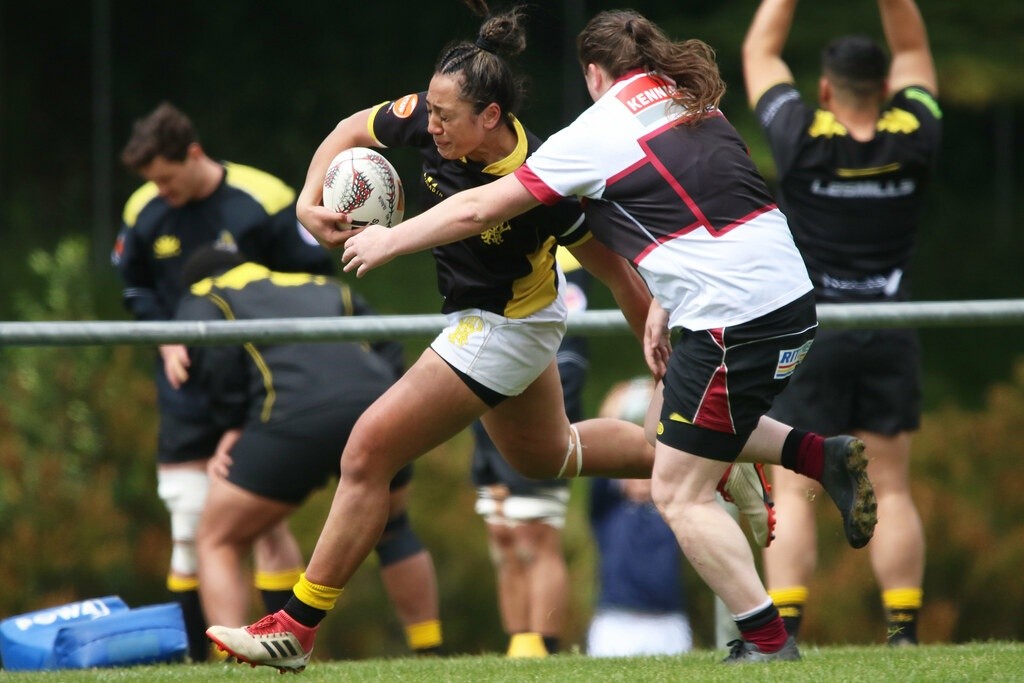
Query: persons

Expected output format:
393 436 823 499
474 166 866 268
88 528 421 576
176 247 448 661
108 107 336 663
206 0 941 676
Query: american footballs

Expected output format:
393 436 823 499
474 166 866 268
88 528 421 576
321 147 404 232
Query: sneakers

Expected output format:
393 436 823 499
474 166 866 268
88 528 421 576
720 639 801 664
722 461 775 546
205 610 322 674
819 434 877 549
